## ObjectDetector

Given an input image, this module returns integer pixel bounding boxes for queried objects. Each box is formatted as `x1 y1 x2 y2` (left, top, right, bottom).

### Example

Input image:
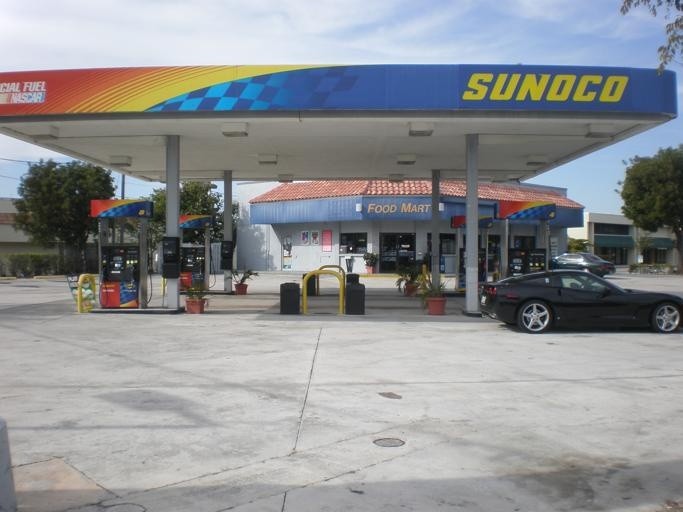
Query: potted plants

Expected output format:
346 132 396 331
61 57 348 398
394 259 423 297
229 267 259 295
182 280 209 313
363 252 378 274
419 279 454 316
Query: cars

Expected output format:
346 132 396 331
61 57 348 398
551 252 616 279
478 269 682 334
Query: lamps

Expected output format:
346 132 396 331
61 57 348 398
507 175 520 182
109 154 132 168
408 120 435 137
584 123 616 140
277 174 293 184
258 153 278 165
389 174 404 183
31 126 58 142
526 155 547 167
220 122 249 137
396 153 417 166
